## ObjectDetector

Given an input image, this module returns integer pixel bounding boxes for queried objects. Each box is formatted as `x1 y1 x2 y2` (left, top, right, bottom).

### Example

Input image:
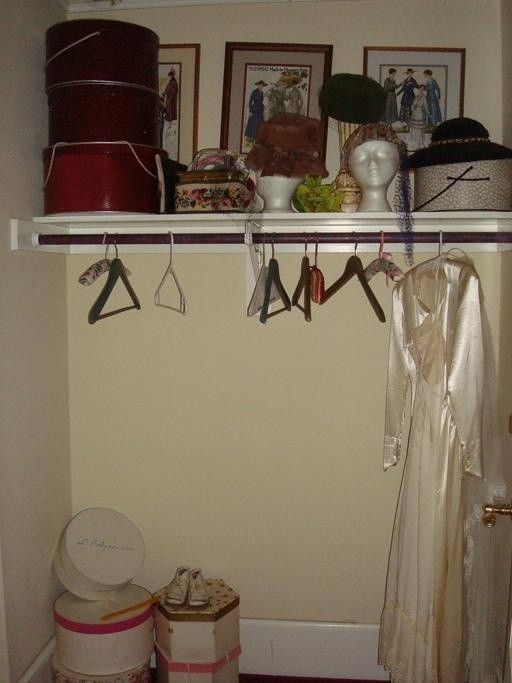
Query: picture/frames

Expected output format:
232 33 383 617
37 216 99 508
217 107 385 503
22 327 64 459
156 42 201 168
219 39 336 167
360 44 468 172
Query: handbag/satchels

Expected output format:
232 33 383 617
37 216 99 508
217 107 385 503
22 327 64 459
169 146 255 215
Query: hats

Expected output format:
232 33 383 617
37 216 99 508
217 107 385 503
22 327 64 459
340 124 406 169
402 112 512 171
254 80 268 88
403 67 416 75
319 71 388 125
244 110 331 177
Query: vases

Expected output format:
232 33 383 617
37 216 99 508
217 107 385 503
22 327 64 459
254 175 306 213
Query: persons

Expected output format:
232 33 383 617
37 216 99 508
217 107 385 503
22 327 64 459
381 66 442 151
344 119 406 213
161 71 178 130
245 77 303 139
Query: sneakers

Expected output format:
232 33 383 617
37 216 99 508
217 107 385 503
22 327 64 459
163 565 210 606
